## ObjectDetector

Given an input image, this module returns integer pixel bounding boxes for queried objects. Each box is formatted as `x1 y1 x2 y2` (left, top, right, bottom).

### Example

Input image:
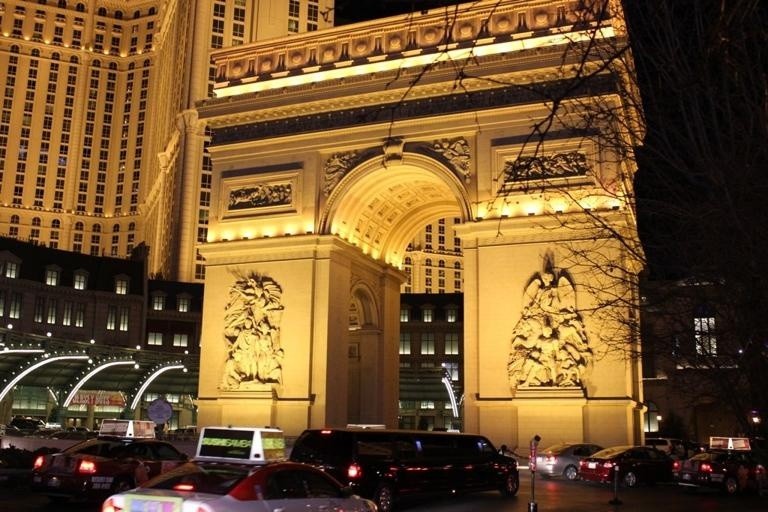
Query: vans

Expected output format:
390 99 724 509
290 429 520 512
174 427 197 441
646 438 683 455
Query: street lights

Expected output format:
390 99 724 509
748 410 762 448
656 415 662 433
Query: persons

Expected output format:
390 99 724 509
222 278 284 391
509 272 589 388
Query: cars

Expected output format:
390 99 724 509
529 443 606 481
580 445 674 487
672 437 768 497
30 420 189 500
6 416 100 441
103 426 378 511
